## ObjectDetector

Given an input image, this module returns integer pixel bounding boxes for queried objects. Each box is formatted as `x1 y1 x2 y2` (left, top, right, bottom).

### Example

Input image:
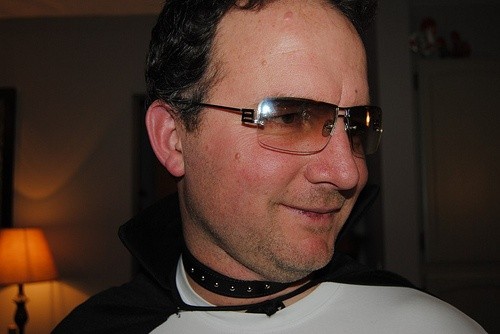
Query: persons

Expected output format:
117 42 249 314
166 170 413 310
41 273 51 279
47 0 488 334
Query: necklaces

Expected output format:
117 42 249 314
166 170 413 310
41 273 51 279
179 237 318 298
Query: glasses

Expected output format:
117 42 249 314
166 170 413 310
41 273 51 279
168 96 383 159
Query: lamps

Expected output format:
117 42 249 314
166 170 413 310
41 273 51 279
0 228 58 334
